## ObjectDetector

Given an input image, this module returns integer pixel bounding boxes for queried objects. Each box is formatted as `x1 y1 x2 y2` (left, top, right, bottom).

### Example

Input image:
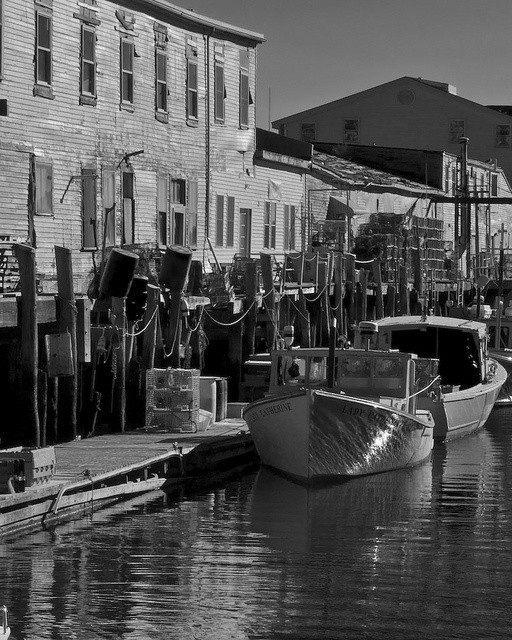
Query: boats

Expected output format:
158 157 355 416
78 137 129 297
352 314 508 442
242 321 434 478
